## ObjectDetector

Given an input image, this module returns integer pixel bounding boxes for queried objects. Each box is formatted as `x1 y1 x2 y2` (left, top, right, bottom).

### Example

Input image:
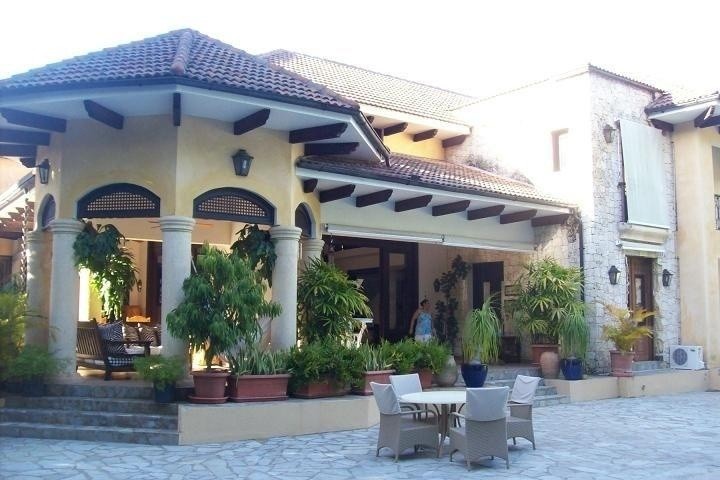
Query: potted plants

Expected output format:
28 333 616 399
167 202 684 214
512 258 584 364
35 158 50 184
460 291 502 387
600 304 659 376
227 347 292 401
288 338 358 398
349 339 397 396
663 269 674 286
554 302 585 379
165 243 283 401
393 338 449 388
70 217 142 322
7 345 69 395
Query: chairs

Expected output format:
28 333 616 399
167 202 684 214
505 374 541 451
369 382 439 462
388 372 442 451
448 386 509 472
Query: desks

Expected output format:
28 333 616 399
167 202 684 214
400 391 466 454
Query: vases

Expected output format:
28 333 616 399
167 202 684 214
437 354 458 385
541 351 560 378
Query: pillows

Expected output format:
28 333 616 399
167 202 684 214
122 325 162 346
99 323 127 354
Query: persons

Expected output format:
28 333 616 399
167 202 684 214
408 298 437 343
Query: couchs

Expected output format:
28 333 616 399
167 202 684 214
119 324 162 354
76 319 152 380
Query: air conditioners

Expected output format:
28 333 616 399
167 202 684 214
670 345 704 371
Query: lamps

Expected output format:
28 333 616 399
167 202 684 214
608 265 621 284
603 124 617 142
231 148 255 176
